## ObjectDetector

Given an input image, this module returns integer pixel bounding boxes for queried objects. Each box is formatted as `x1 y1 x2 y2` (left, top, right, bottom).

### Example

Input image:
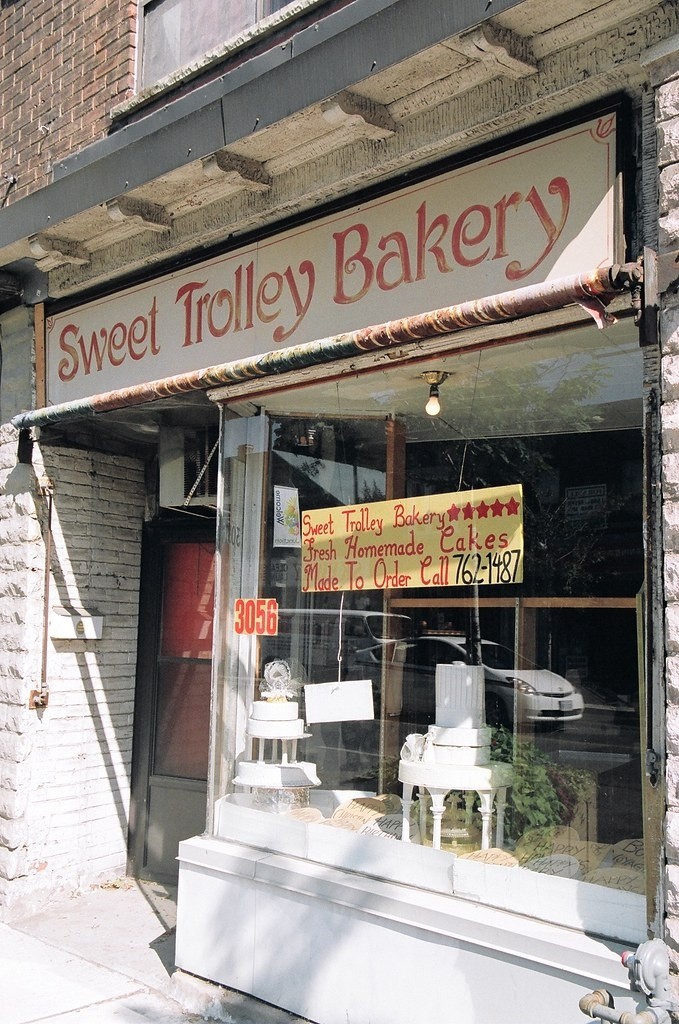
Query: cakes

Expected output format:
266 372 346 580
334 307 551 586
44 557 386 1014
247 701 304 738
236 758 316 786
427 724 491 766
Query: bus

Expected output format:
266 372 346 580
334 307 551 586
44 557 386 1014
258 607 413 682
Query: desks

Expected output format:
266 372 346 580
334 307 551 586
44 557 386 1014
397 759 514 853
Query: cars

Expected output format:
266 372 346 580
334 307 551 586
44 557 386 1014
354 631 585 729
